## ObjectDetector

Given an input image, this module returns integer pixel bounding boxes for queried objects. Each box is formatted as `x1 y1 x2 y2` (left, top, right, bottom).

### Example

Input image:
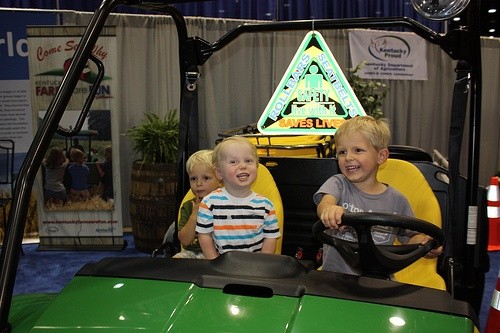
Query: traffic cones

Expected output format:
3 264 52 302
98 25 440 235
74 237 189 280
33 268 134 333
487 176 500 252
482 271 500 333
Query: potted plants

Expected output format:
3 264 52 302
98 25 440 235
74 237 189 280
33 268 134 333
119 108 180 255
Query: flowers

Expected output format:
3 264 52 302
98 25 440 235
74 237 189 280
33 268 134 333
346 60 391 118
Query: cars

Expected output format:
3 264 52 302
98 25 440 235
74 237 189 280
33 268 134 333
0 0 487 333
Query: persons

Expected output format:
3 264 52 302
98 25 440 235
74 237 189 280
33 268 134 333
172 149 226 259
311 115 445 280
194 134 281 260
37 138 114 207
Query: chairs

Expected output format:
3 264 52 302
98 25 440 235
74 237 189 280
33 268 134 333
178 163 284 255
317 159 446 291
